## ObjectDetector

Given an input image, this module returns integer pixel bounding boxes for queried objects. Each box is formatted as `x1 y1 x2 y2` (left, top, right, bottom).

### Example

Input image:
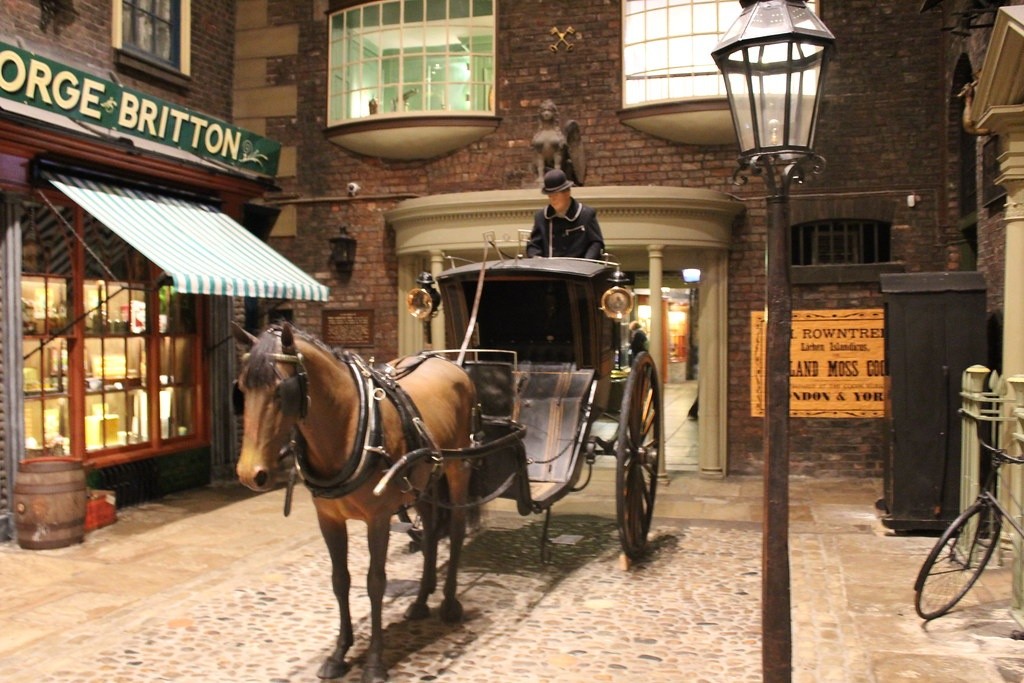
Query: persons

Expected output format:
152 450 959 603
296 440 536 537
628 321 646 356
532 99 567 162
689 397 698 419
527 170 605 259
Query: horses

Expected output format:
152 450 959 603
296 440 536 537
228 319 479 682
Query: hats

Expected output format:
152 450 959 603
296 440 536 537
541 169 574 194
629 321 640 329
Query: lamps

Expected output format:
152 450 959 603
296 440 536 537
327 226 357 265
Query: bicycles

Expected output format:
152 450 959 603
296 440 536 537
913 438 1024 621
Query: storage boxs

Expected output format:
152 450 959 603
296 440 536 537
84 488 118 533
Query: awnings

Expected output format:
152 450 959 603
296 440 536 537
36 170 330 302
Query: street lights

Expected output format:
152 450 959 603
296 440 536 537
710 1 838 683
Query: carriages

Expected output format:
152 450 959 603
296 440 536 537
229 228 666 682
